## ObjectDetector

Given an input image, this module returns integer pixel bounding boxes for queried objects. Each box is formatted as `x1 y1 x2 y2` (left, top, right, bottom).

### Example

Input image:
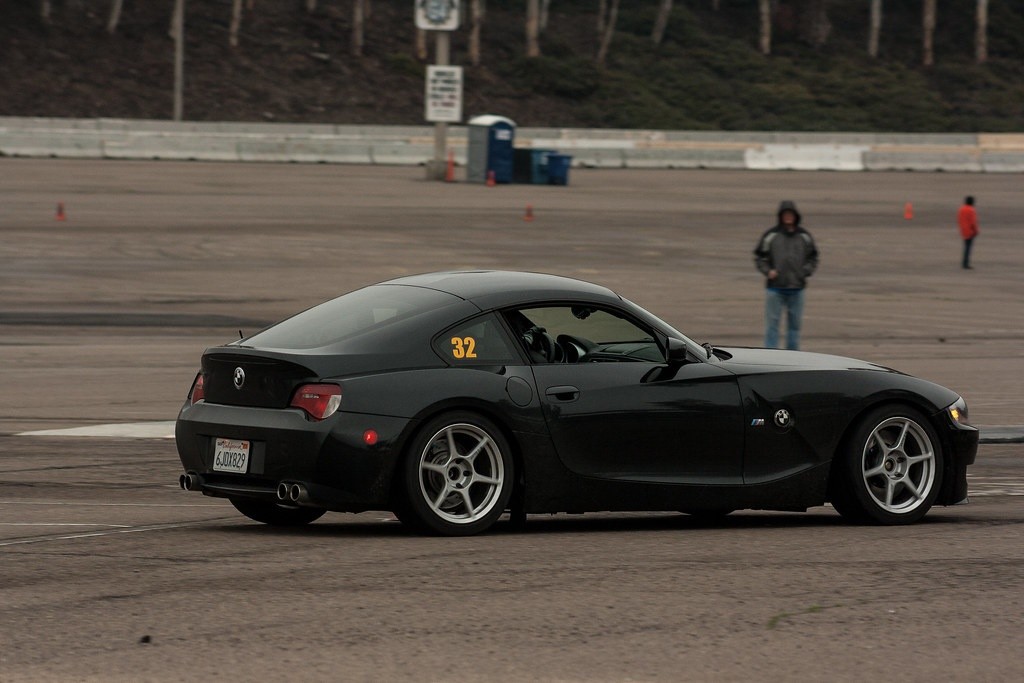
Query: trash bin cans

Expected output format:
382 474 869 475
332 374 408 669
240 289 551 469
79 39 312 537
547 155 572 185
467 115 516 184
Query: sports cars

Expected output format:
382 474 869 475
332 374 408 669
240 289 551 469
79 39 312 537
172 267 981 539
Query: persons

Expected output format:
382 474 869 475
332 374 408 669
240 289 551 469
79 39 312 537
753 199 820 351
959 194 982 270
522 324 547 351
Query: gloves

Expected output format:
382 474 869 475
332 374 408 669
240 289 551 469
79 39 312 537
524 325 547 341
